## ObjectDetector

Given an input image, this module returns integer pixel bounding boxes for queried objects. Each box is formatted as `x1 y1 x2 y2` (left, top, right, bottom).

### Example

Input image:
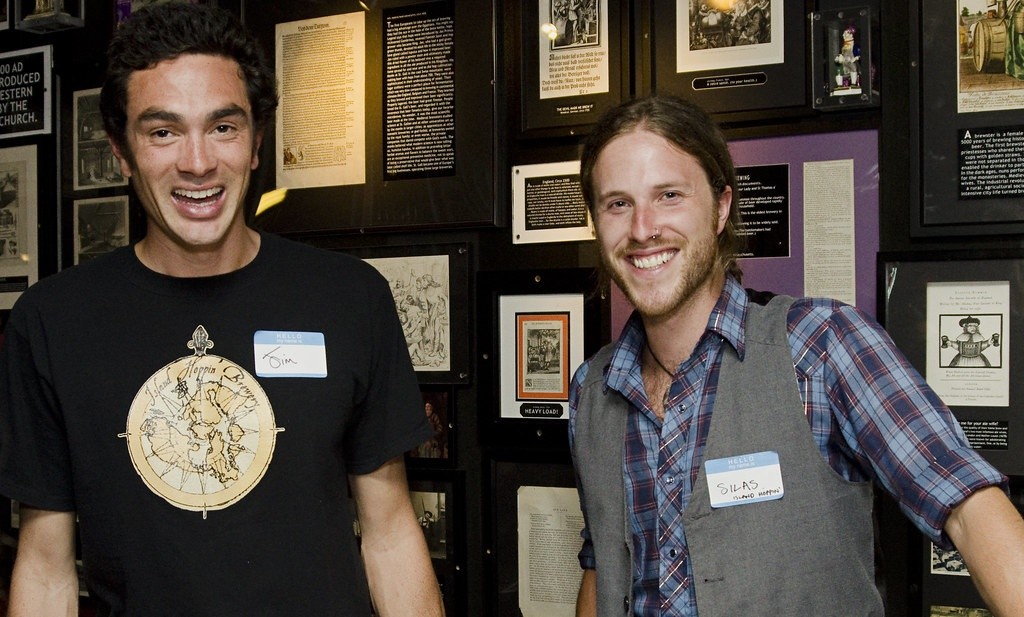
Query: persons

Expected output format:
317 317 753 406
567 97 1024 617
0 0 448 617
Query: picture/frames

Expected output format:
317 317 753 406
380 385 466 617
476 264 610 446
326 240 480 385
876 247 1024 482
906 475 1024 617
652 0 804 131
518 1 631 142
806 1 881 114
909 0 1024 237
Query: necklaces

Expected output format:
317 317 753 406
643 330 676 376
